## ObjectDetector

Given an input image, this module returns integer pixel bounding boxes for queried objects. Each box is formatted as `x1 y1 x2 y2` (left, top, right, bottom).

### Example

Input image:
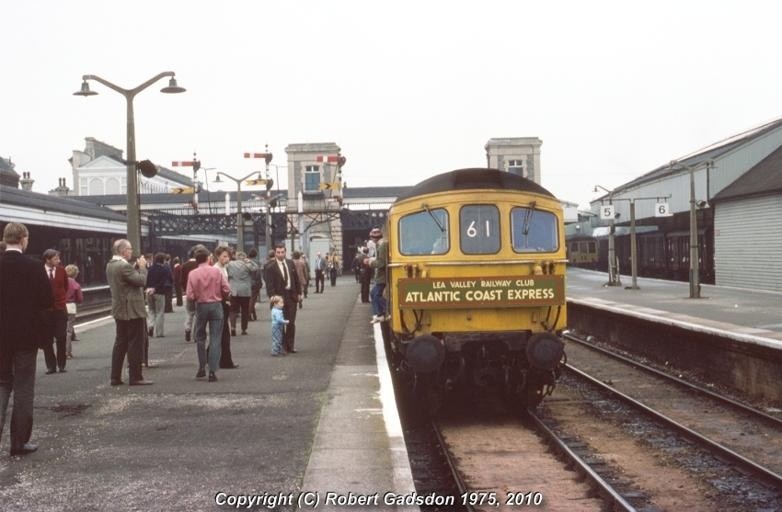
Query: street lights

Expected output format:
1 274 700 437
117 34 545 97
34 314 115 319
662 157 718 299
248 192 284 255
212 170 264 253
72 68 188 268
593 184 621 286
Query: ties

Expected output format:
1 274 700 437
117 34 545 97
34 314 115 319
49 269 54 281
282 262 288 282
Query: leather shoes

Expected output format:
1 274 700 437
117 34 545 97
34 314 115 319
11 444 38 458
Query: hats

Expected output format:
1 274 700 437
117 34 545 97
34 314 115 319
369 225 383 237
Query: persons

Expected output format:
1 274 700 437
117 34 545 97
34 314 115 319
325 253 331 280
105 238 156 388
1 221 56 455
350 228 391 324
142 236 311 381
41 248 69 374
330 248 340 287
314 251 327 294
64 263 85 356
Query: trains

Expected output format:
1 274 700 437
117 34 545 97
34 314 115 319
0 182 254 318
381 167 572 420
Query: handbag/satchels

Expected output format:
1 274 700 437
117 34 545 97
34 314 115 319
65 302 76 315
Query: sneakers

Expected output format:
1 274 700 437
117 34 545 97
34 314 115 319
111 377 153 385
186 327 248 382
370 313 385 324
148 326 154 336
249 312 257 321
46 337 80 374
